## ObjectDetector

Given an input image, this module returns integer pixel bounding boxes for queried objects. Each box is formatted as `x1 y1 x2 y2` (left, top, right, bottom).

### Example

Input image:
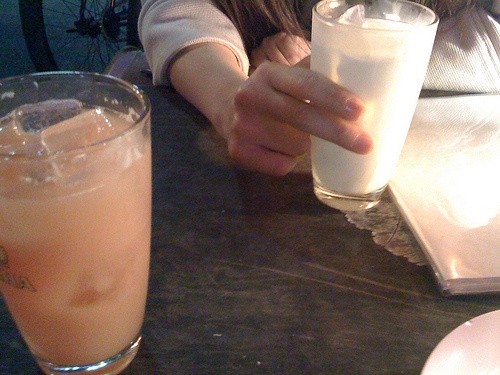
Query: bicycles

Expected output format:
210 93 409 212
19 0 144 83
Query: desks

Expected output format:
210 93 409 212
0 43 500 375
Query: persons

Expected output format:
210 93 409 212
136 0 500 179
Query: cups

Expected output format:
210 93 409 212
0 71 152 375
310 0 440 211
